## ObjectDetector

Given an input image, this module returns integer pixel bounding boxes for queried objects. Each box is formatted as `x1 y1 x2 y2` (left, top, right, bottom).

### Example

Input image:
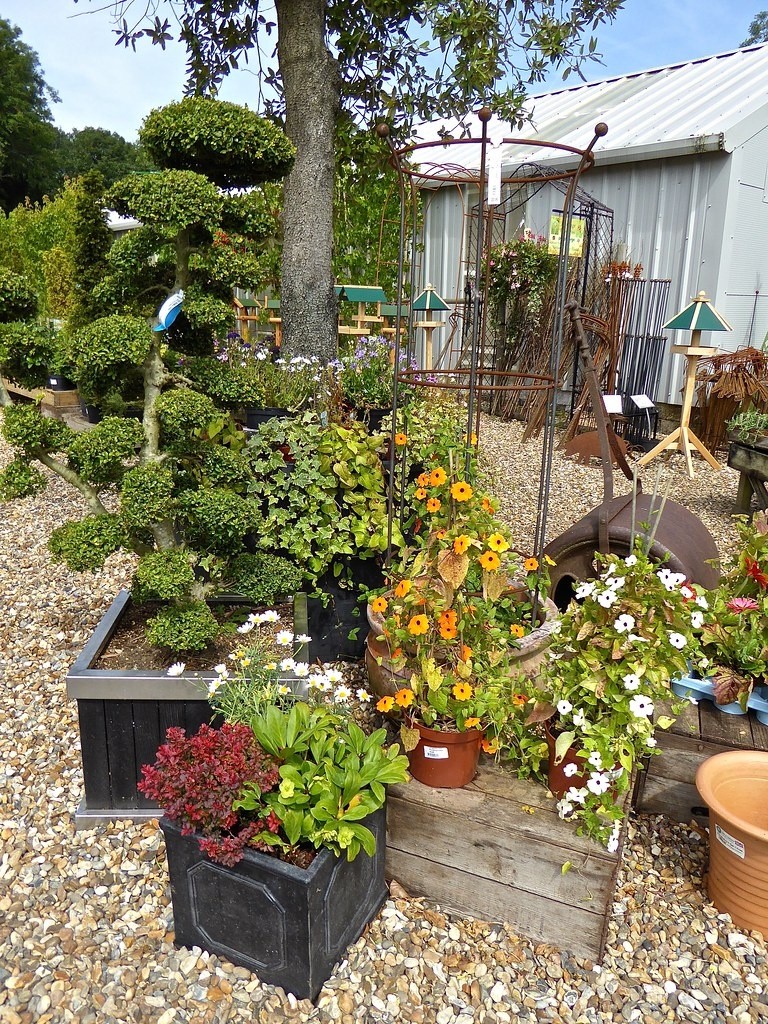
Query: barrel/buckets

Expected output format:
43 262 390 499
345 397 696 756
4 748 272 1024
695 749 768 944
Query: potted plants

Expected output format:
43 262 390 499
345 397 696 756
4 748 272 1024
0 168 313 822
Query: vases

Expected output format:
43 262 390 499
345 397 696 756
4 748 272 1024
693 748 768 942
400 708 481 789
366 587 561 727
159 790 391 1004
544 720 623 806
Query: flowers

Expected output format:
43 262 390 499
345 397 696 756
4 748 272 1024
366 422 768 852
128 608 412 868
